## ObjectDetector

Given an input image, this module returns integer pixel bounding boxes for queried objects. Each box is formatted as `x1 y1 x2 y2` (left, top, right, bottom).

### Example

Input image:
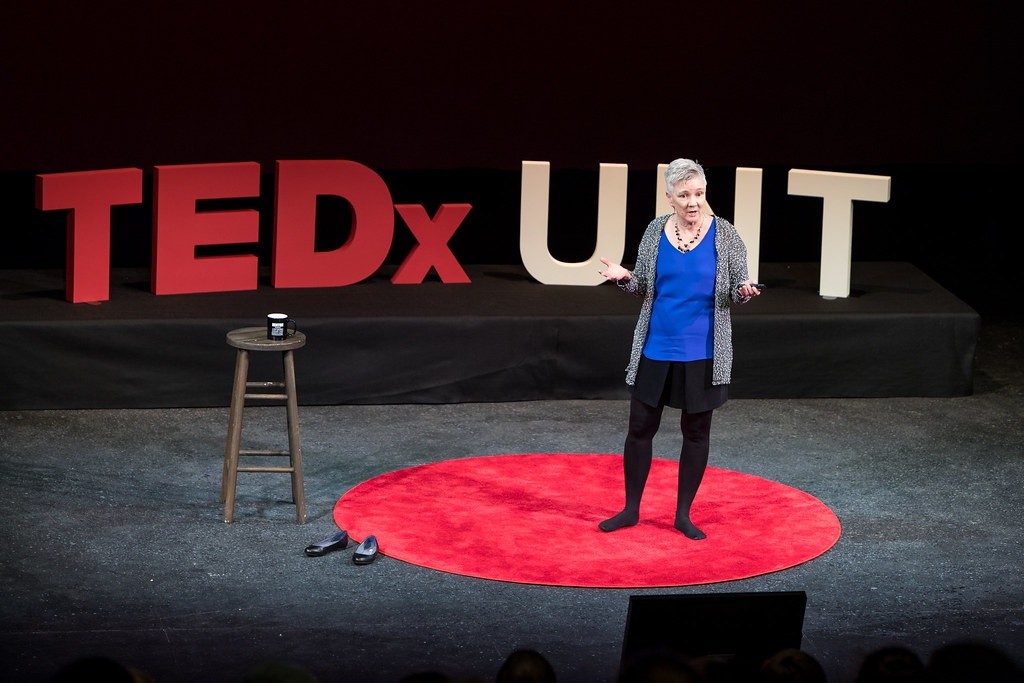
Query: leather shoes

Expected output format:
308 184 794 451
353 536 378 565
305 531 348 557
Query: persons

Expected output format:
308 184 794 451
598 158 761 539
497 649 558 683
619 649 701 683
760 649 827 683
928 641 1024 683
854 645 927 683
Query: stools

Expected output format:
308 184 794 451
220 324 309 524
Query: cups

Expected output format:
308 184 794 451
266 312 297 341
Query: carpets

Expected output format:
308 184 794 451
334 451 841 588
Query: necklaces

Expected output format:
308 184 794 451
672 214 703 252
